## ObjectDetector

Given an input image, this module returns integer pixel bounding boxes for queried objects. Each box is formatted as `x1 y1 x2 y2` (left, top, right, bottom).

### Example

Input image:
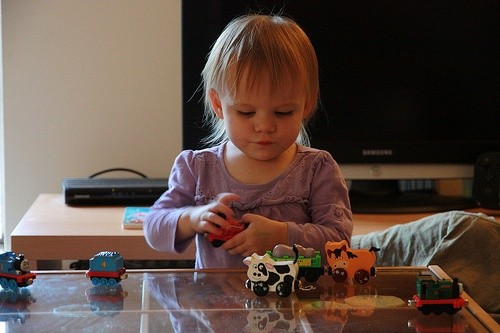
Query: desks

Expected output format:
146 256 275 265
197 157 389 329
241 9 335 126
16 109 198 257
0 266 500 333
12 194 500 271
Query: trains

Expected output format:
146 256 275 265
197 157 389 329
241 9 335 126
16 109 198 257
406 274 466 316
0 251 37 290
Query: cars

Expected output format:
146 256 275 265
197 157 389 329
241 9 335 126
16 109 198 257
242 252 303 298
324 240 381 285
204 208 247 248
265 243 324 283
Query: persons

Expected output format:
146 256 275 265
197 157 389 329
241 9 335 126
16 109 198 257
144 16 354 269
145 273 349 333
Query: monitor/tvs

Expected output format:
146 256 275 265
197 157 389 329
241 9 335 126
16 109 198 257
180 0 500 214
